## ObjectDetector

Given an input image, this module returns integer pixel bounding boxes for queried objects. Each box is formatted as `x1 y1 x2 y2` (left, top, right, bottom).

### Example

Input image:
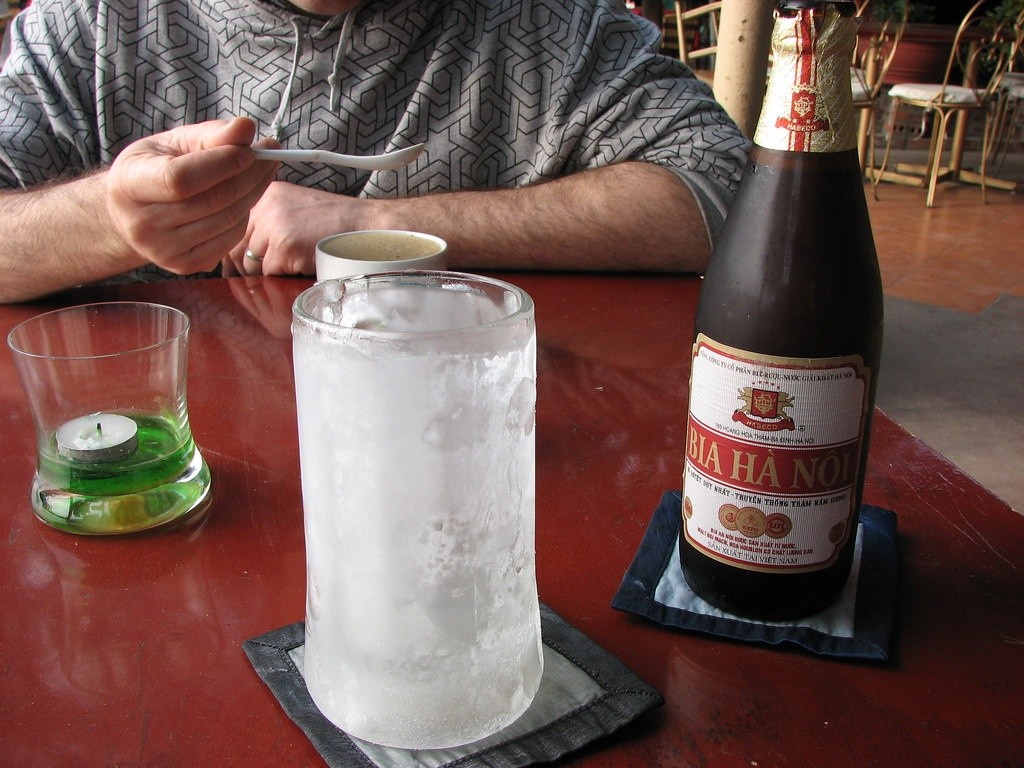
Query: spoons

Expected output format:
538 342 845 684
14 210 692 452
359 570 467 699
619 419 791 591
251 142 424 170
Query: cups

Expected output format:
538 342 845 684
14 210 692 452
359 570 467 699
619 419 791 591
315 230 448 285
291 270 543 750
6 300 213 537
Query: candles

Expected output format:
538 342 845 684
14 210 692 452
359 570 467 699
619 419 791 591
56 413 139 462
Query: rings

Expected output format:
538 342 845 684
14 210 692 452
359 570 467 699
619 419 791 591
248 286 259 295
245 247 264 262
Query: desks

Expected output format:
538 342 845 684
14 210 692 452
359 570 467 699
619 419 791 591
0 269 1024 768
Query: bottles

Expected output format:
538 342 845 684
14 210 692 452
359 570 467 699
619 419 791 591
679 0 886 624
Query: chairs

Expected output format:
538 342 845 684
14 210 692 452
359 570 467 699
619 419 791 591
850 0 1024 207
675 0 722 89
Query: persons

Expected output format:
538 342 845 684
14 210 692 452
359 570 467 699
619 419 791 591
0 0 751 303
0 278 316 436
